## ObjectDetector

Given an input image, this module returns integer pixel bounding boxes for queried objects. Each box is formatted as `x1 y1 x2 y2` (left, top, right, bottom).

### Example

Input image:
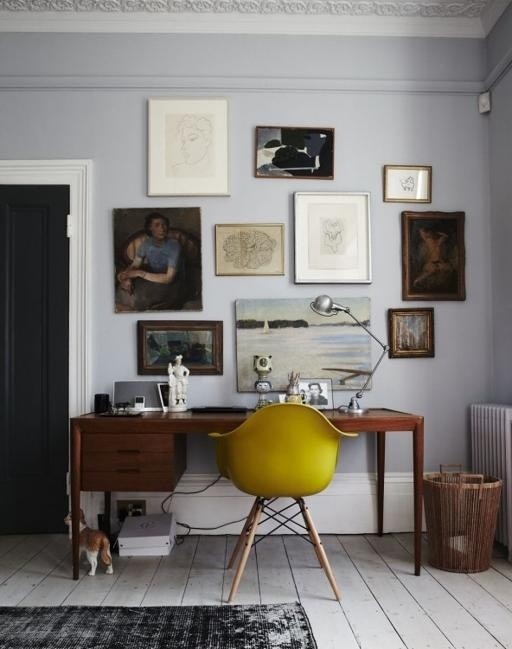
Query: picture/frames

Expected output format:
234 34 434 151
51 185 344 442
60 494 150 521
382 164 432 204
402 209 468 301
138 319 226 377
256 123 335 181
300 377 335 411
146 97 233 198
388 307 431 360
215 222 286 276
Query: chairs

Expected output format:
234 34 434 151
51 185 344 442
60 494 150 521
210 406 361 605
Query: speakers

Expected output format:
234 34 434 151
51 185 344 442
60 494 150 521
95 394 109 414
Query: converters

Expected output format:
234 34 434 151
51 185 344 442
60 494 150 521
133 511 142 517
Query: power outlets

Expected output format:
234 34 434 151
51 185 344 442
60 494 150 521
128 505 143 515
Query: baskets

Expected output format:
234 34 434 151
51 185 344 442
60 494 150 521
421 462 502 574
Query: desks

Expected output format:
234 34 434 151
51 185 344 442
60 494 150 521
69 405 424 581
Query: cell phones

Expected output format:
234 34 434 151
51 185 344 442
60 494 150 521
134 395 145 408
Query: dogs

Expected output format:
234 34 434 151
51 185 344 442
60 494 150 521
63 508 114 576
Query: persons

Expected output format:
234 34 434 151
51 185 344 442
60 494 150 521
308 382 327 404
117 213 190 307
413 220 455 287
168 354 190 405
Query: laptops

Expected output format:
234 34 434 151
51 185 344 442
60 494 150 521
191 407 248 413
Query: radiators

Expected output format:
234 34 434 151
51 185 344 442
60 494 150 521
468 401 512 568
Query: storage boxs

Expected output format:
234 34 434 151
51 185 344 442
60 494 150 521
116 512 178 556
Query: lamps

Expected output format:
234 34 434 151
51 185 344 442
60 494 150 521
311 295 390 412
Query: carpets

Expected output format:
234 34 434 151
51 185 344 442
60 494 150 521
3 602 315 649
